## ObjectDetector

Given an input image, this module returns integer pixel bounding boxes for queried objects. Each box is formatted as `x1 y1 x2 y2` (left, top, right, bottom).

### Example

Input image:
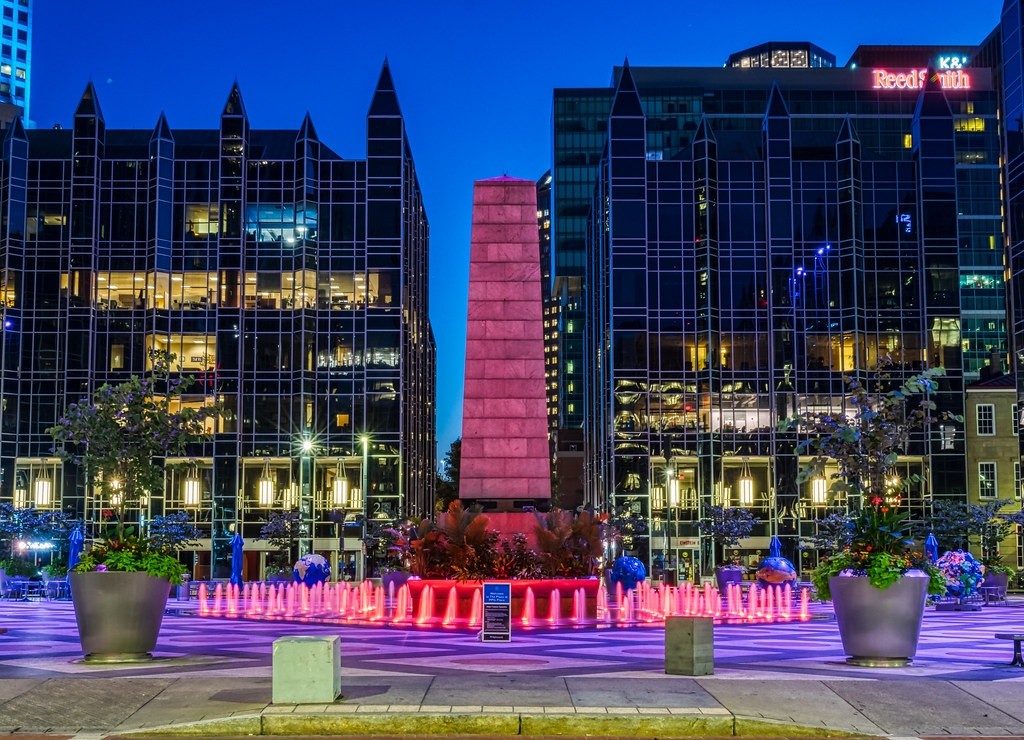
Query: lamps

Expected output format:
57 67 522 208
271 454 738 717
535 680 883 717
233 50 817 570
333 458 348 505
184 467 199 505
35 458 50 507
258 458 274 505
738 458 753 507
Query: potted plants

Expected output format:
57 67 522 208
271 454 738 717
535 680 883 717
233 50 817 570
692 501 762 597
358 506 412 592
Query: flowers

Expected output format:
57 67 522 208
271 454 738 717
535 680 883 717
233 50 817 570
934 550 986 600
806 494 949 601
69 508 192 586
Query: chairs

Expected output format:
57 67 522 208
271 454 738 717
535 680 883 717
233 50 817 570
40 581 56 602
981 573 1008 606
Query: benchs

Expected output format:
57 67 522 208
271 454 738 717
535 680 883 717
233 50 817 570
995 634 1024 667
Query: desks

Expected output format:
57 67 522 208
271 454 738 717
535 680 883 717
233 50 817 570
11 581 40 602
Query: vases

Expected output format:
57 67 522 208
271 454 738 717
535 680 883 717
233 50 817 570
68 568 173 664
827 569 931 668
